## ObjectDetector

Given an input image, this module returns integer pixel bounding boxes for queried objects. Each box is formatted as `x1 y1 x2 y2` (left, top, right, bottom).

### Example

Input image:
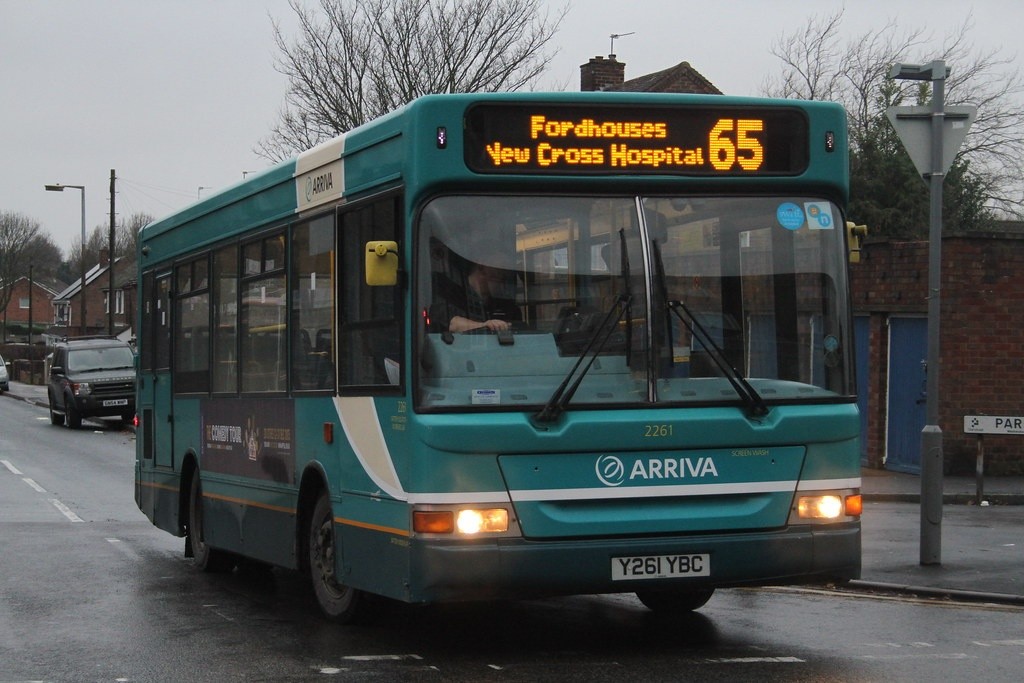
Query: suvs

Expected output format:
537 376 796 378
45 334 135 429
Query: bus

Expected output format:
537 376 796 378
132 87 872 624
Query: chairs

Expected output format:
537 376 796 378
183 306 620 394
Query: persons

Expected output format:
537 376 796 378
428 244 524 333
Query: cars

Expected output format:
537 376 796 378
0 354 12 396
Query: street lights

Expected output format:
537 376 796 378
43 184 87 334
888 57 948 566
